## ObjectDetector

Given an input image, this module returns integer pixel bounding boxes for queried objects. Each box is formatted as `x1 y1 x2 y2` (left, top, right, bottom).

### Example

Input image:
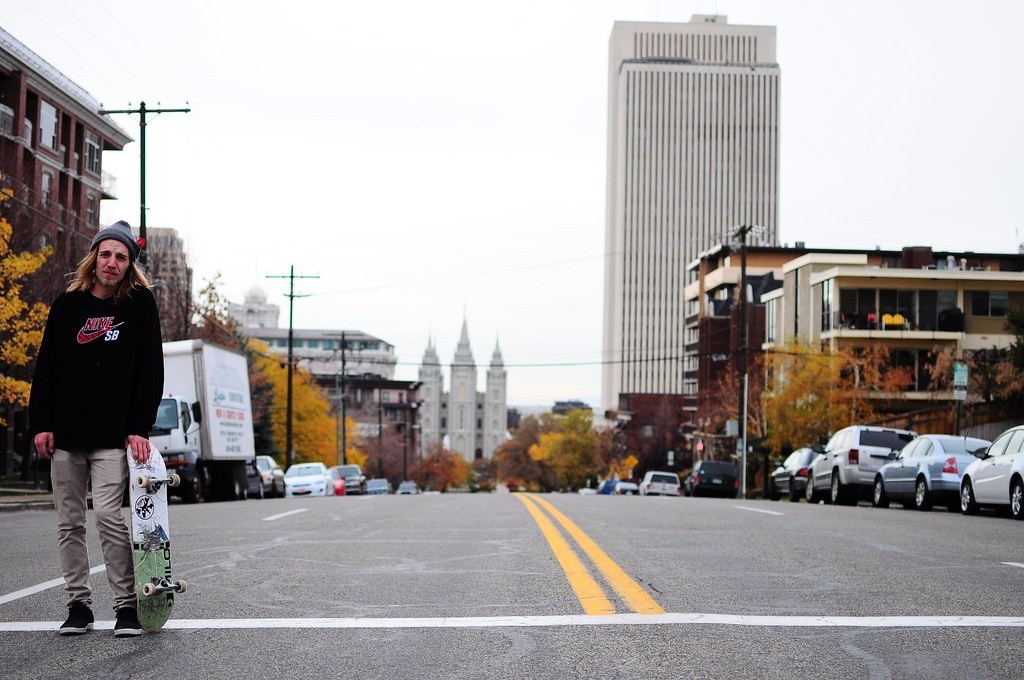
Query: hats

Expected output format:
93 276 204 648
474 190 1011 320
90 220 140 263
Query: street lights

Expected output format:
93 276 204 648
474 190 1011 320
760 341 860 426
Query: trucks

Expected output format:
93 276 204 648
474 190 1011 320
120 337 256 504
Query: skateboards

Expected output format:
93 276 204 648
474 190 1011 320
125 441 188 634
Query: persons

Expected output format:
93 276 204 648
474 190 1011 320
28 221 165 637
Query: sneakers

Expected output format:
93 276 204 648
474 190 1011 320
60 605 95 633
113 607 147 635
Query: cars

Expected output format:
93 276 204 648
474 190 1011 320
638 470 680 498
285 461 335 498
871 433 993 512
398 480 418 495
327 464 368 495
254 455 287 498
367 478 390 495
246 460 265 500
959 424 1024 521
768 442 826 502
577 479 638 495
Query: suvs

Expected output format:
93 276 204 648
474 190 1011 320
805 424 921 505
684 459 738 499
